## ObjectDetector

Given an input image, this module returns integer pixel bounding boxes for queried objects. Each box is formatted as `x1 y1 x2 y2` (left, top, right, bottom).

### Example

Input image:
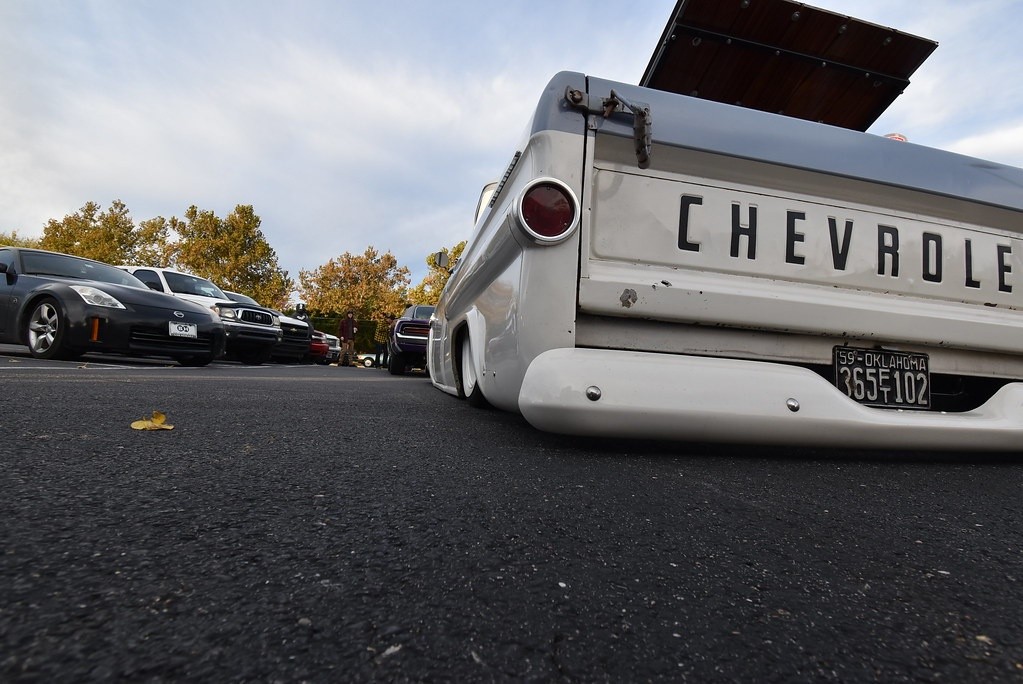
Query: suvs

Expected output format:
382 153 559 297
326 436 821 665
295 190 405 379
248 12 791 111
81 264 283 365
195 286 309 364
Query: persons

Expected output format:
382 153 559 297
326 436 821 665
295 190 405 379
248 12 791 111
374 313 396 368
338 310 358 367
292 303 308 322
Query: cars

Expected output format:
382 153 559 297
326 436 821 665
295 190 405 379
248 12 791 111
316 333 341 365
307 330 328 358
387 306 435 375
0 246 226 367
356 352 390 367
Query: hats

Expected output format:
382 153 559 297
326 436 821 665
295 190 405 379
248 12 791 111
347 311 353 314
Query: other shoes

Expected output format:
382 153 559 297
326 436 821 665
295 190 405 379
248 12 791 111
376 366 381 369
382 365 389 368
338 363 343 366
349 365 357 367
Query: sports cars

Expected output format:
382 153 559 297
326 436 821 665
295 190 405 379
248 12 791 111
427 1 1023 462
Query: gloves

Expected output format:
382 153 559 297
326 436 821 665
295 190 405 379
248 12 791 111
353 326 358 331
340 337 344 341
